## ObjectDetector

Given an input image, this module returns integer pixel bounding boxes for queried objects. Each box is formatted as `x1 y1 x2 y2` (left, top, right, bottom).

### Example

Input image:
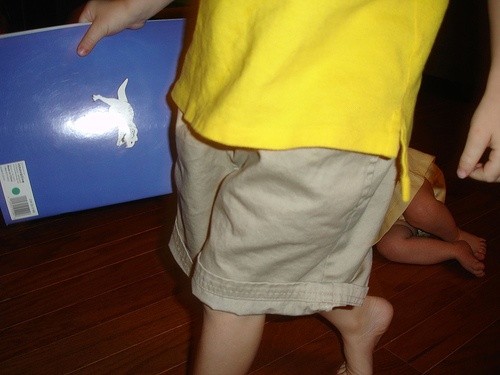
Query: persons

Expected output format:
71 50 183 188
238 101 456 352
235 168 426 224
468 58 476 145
78 0 499 375
373 145 486 278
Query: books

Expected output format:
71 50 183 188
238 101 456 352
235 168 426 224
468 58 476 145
1 17 186 226
